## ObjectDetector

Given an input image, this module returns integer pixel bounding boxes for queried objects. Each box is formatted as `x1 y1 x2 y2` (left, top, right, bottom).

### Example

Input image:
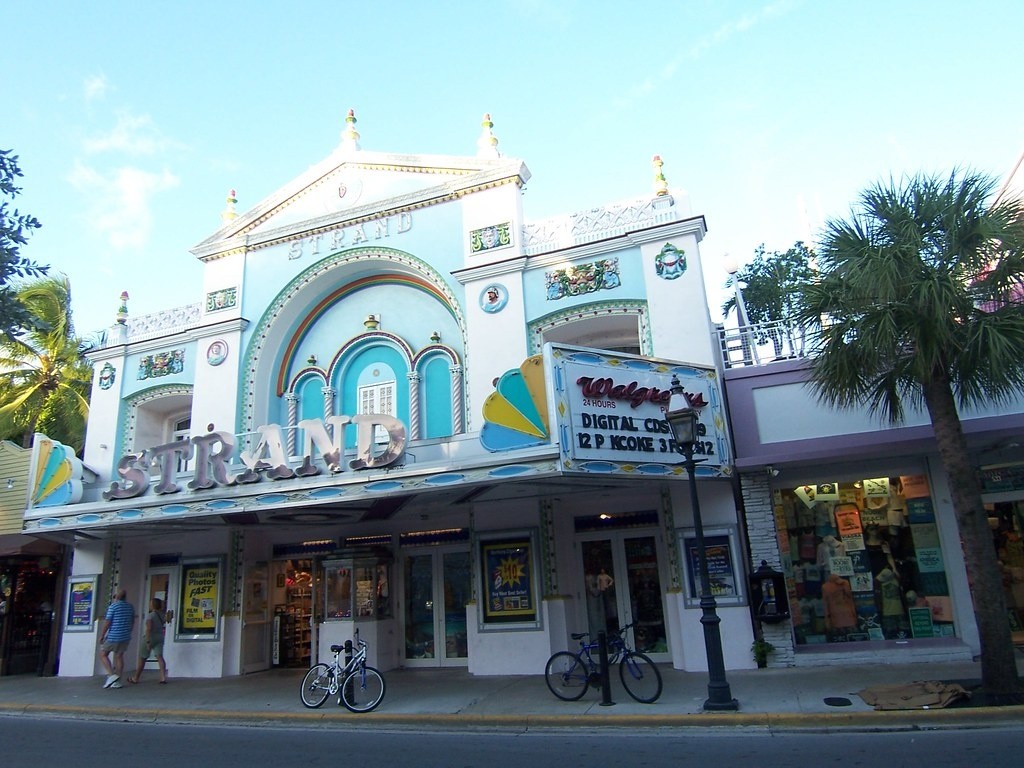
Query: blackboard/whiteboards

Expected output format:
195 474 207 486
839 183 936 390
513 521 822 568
908 607 934 638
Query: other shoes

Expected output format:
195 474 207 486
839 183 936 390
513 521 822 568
102 674 121 689
127 676 137 684
110 681 124 688
158 680 167 684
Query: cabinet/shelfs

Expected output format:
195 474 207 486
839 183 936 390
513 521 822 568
288 592 318 666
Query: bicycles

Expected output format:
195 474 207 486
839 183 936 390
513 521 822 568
544 620 662 704
300 627 387 714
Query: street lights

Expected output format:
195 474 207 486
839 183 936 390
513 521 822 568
664 374 742 711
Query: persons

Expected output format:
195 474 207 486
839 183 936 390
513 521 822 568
597 568 613 590
865 522 943 637
127 598 168 684
99 589 135 688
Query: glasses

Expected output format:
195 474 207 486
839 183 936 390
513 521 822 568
113 593 116 599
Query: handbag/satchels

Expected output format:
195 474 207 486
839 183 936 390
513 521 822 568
162 627 166 636
